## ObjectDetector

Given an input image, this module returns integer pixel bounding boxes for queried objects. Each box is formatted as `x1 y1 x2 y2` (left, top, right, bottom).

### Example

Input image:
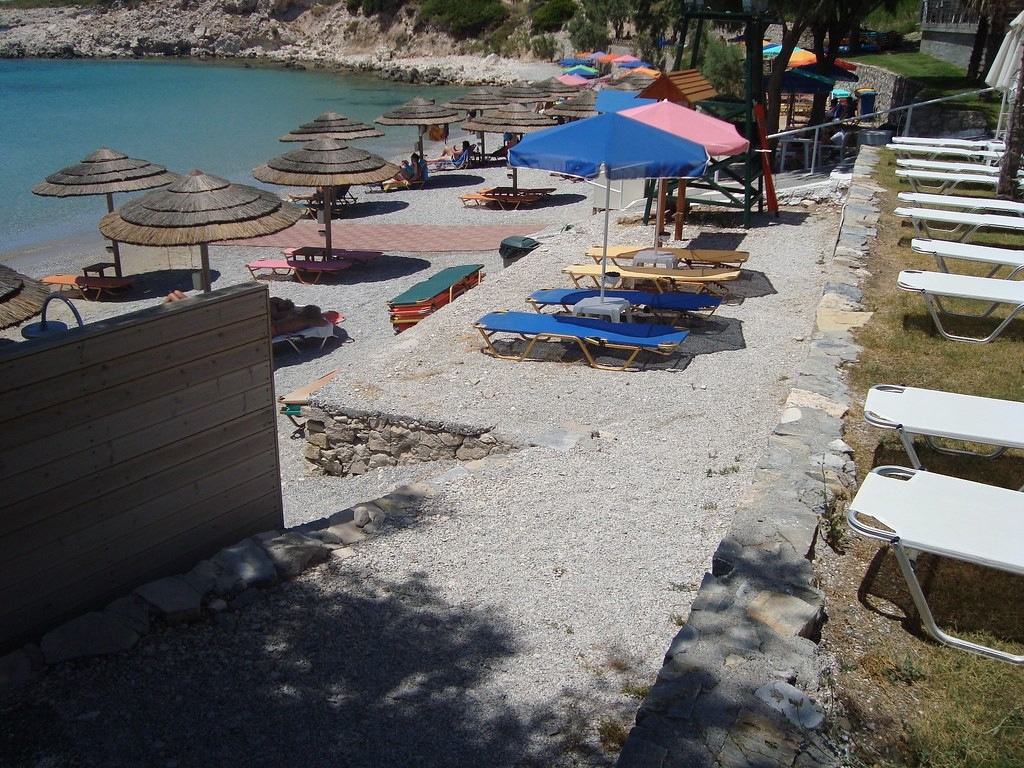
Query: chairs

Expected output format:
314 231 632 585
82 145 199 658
896 268 1024 343
244 260 352 285
893 207 1024 248
292 203 343 221
478 187 557 200
428 144 479 171
818 132 852 167
365 161 428 192
895 169 1024 198
480 137 521 162
291 185 360 209
885 144 1024 166
896 158 1024 178
282 250 382 266
458 194 541 211
39 275 134 299
911 237 1024 280
473 310 690 372
892 136 1007 160
271 305 345 355
387 263 486 337
280 369 339 438
586 244 750 270
898 192 1024 232
525 287 724 326
846 467 1024 666
822 105 850 130
562 263 741 297
861 382 1024 489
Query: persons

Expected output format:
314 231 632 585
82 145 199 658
788 90 859 153
625 32 632 39
287 97 570 197
665 35 677 44
163 289 328 338
718 35 726 44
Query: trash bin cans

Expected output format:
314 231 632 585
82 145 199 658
497 235 543 268
854 87 878 123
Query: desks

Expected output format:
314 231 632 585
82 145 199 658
630 251 677 291
484 187 517 197
83 262 116 280
292 247 328 260
573 296 632 322
779 137 813 173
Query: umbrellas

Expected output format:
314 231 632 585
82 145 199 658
727 35 858 123
0 265 51 329
251 76 602 195
985 11 1024 142
508 98 750 320
557 53 662 93
98 171 303 292
32 149 179 278
